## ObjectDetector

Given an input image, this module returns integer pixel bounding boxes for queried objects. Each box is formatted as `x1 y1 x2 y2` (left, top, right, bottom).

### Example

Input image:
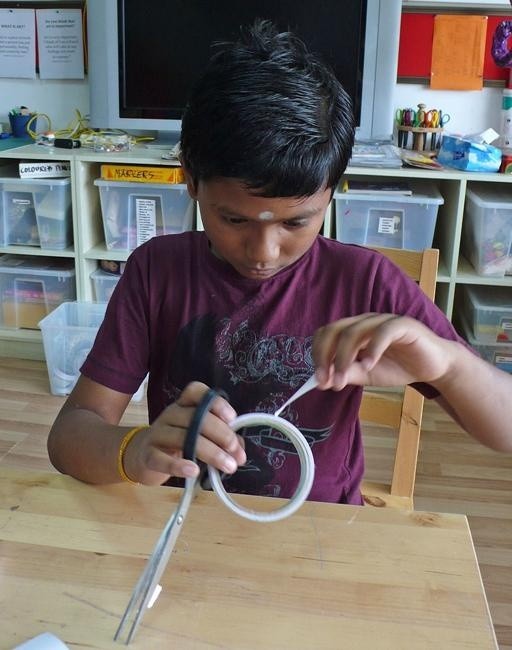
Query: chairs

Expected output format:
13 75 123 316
354 248 439 510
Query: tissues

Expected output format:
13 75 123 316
439 127 504 173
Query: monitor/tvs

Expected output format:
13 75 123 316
118 0 382 139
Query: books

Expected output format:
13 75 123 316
342 178 413 196
3 297 48 303
5 286 63 301
392 146 445 171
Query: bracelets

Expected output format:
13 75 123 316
117 423 151 487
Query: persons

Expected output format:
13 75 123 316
46 20 512 491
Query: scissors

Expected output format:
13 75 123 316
396 108 450 151
114 387 247 646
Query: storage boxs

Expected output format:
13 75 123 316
1 254 74 331
90 268 120 303
37 302 148 401
2 178 70 251
333 176 511 369
94 180 192 253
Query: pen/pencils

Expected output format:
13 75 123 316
9 106 32 116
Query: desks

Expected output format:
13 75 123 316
1 463 500 650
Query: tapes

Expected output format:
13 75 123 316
207 362 336 522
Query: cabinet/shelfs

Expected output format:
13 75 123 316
1 149 512 342
